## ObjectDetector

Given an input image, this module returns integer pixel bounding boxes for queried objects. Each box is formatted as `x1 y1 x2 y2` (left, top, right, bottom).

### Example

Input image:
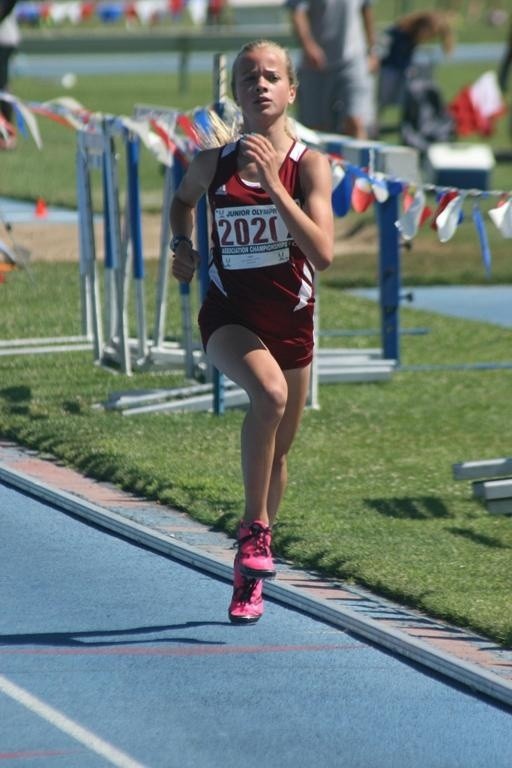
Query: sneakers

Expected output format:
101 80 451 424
227 552 265 626
236 516 277 580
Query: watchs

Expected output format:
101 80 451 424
169 232 192 251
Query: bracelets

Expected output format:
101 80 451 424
366 48 381 53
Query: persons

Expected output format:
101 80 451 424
0 0 20 141
287 4 379 145
377 8 452 140
170 42 335 626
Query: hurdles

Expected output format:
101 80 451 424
91 112 254 419
302 131 414 412
0 102 99 357
